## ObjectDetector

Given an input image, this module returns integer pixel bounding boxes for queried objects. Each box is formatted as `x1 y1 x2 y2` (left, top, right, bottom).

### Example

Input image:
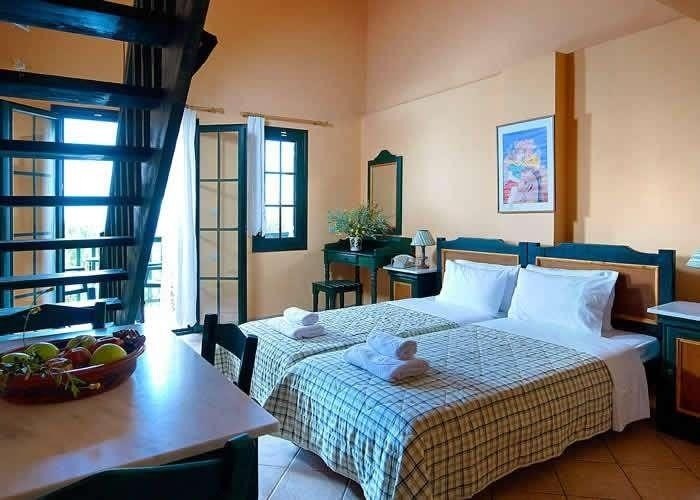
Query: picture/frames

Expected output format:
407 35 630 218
495 114 556 213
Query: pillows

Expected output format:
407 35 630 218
439 259 620 336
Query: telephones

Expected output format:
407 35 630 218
392 254 416 269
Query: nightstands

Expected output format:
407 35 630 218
647 301 700 444
383 265 438 301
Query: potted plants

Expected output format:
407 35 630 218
327 199 393 252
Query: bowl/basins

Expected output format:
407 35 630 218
0 336 144 407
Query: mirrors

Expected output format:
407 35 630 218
367 149 403 235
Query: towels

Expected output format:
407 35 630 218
265 315 325 339
343 342 426 383
365 329 418 360
282 306 320 326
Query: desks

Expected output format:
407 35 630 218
322 237 412 310
0 326 280 500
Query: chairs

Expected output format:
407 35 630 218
201 314 258 396
33 434 259 499
1 302 106 336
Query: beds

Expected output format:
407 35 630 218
262 243 676 500
215 237 527 405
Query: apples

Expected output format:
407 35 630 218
24 342 60 362
44 358 73 373
65 335 96 352
92 337 124 353
1 352 32 372
64 347 91 368
90 343 127 366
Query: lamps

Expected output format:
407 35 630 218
685 247 700 269
411 230 435 269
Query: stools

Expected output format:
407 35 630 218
312 280 362 312
